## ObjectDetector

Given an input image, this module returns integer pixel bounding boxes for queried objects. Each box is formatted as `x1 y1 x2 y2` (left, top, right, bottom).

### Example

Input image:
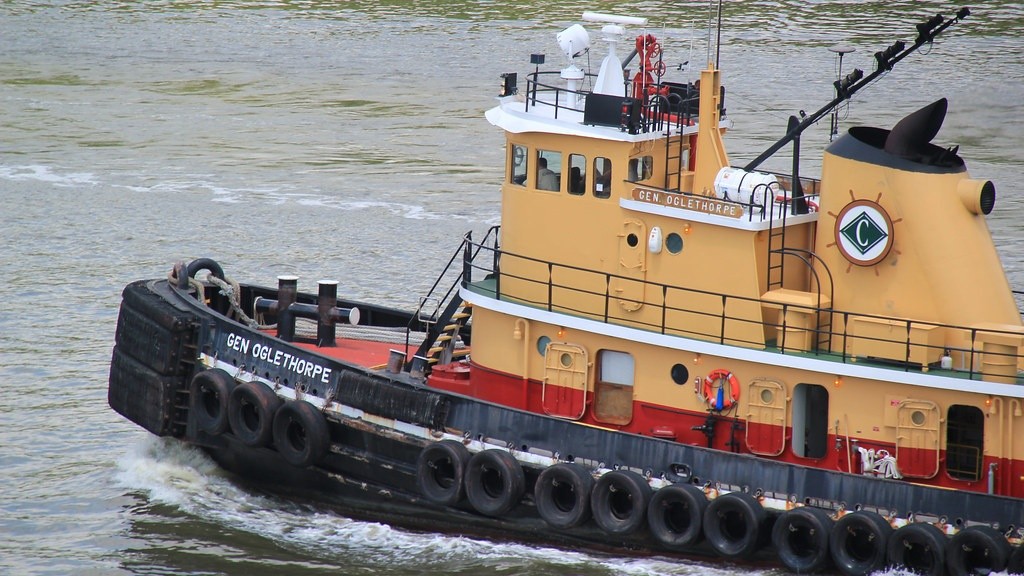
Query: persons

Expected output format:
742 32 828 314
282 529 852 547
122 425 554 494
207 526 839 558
521 158 560 191
576 164 636 195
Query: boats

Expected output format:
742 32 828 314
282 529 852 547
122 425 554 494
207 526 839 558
109 2 1024 575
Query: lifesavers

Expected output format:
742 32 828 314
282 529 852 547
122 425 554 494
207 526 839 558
703 368 741 411
189 368 332 470
416 438 1024 576
